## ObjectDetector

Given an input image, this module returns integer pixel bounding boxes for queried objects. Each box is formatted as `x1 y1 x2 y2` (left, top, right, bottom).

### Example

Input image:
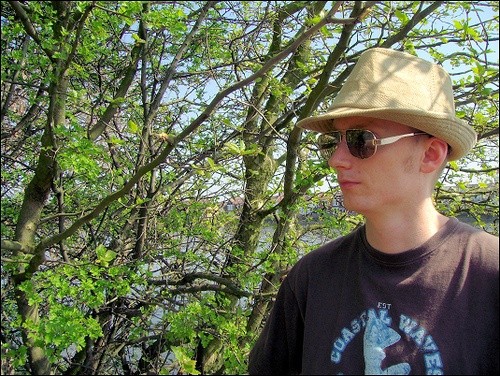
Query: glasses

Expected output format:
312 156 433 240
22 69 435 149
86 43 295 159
318 129 429 160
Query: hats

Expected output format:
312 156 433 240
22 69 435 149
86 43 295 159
296 48 477 162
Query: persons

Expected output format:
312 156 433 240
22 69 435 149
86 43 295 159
246 48 499 375
